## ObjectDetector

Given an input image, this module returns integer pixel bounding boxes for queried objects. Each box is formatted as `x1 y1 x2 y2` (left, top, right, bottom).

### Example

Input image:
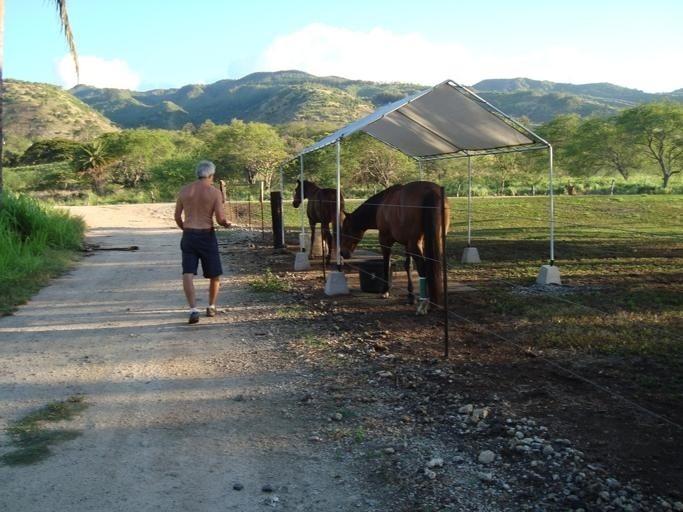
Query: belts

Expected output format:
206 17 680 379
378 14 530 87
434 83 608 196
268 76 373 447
183 227 215 233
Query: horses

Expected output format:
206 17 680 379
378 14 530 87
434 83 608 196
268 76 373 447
339 181 451 317
292 179 346 260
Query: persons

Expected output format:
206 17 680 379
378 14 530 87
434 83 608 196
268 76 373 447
173 160 231 324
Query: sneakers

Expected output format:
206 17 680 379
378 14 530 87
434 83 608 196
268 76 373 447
206 306 218 317
188 311 200 324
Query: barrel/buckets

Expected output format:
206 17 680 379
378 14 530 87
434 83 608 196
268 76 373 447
307 228 328 256
360 258 392 293
